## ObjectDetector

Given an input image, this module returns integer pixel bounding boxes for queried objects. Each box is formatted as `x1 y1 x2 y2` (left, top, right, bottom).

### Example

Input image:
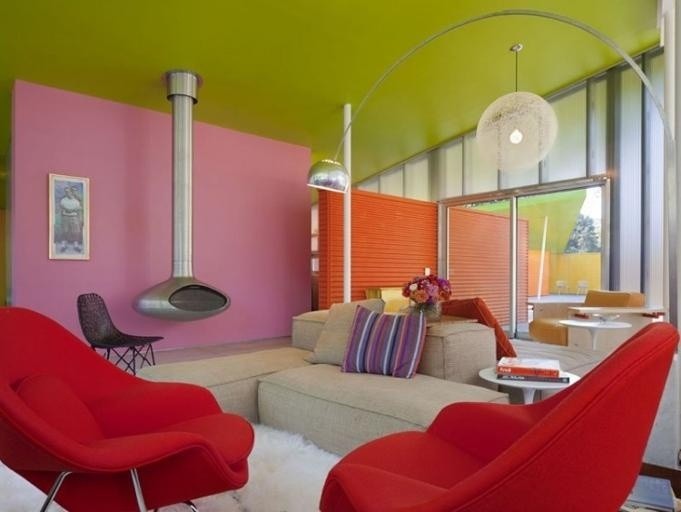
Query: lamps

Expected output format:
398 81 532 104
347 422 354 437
476 44 557 169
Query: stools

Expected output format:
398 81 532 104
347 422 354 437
257 363 518 461
132 347 311 426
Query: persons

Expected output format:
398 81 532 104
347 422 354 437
59 184 82 246
61 186 81 253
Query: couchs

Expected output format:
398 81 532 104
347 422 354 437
292 298 605 397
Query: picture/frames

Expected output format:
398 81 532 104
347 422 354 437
46 173 91 262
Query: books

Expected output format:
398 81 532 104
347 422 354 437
495 357 559 377
497 374 569 384
619 476 677 512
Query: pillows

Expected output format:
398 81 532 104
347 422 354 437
340 304 428 377
438 297 515 358
305 298 384 365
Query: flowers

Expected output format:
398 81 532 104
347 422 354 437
402 273 453 312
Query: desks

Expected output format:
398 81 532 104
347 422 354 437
559 319 634 348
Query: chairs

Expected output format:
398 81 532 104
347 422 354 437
529 290 646 342
305 160 352 192
318 321 679 510
76 291 162 376
1 308 253 512
